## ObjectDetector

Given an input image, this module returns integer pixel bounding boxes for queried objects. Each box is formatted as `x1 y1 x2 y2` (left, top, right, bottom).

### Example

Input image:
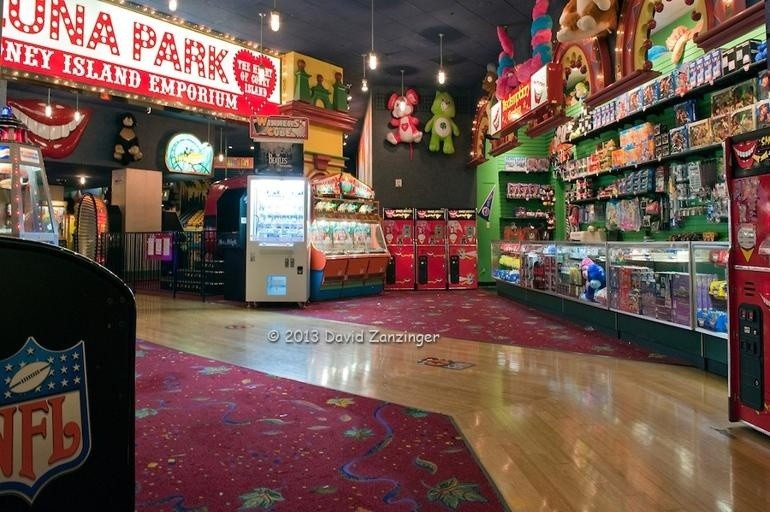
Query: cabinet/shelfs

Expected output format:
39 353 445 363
490 100 569 320
566 19 768 378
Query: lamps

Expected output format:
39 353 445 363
258 0 447 112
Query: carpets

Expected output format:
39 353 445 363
250 285 698 368
135 337 510 512
